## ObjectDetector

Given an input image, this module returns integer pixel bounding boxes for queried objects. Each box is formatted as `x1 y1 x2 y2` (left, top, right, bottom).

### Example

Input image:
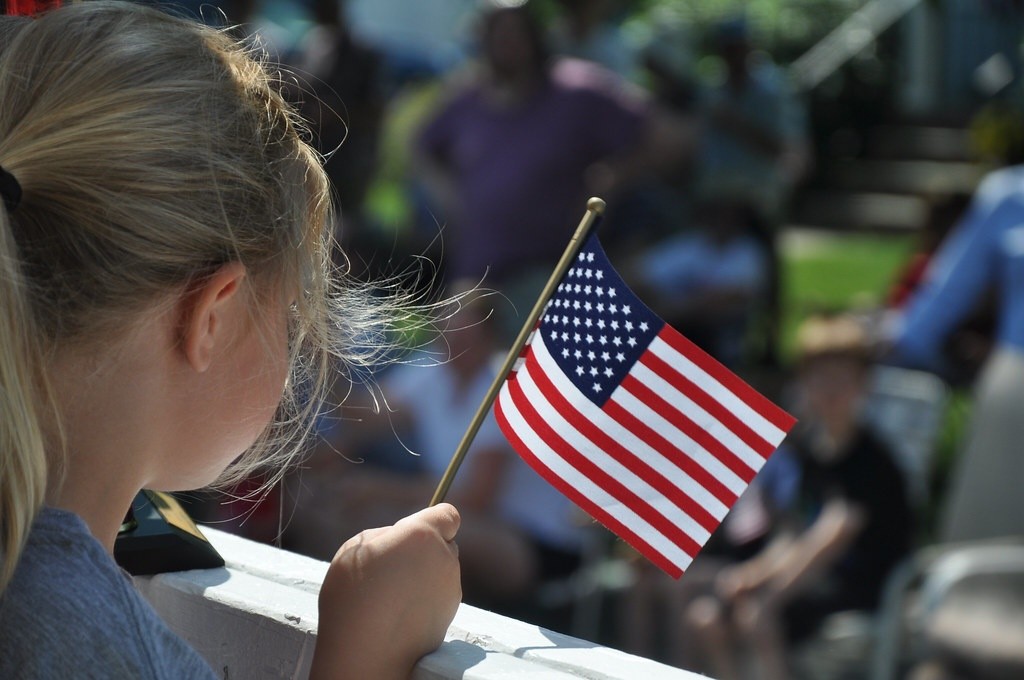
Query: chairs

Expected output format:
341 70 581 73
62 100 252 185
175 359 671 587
793 362 945 680
887 341 1023 680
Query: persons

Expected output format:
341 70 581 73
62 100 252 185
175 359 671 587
968 80 1022 176
272 3 465 250
878 184 978 324
630 338 802 678
292 153 431 287
618 188 775 322
0 3 478 680
631 39 744 236
199 0 262 52
927 321 994 530
690 14 810 242
379 0 693 358
528 0 633 88
878 111 1024 579
181 453 282 544
692 311 915 680
294 259 615 629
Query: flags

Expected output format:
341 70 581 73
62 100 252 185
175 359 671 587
489 231 799 581
